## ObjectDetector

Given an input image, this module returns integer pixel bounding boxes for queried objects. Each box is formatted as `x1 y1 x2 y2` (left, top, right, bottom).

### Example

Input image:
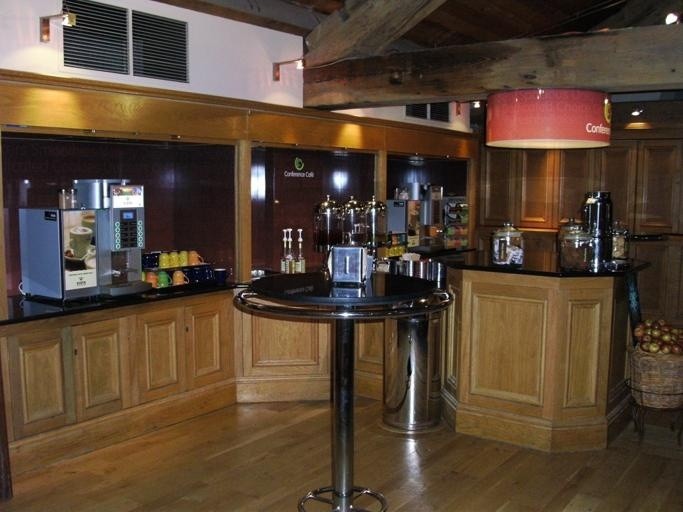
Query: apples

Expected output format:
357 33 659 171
634 318 683 354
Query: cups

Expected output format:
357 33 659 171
142 250 233 290
67 225 94 259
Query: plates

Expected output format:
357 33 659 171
64 245 96 262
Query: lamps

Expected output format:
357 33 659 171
39 12 76 44
272 58 305 81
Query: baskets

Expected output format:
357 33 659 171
628 349 683 409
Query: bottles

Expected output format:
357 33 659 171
490 220 526 267
59 189 79 210
310 194 391 253
559 217 632 269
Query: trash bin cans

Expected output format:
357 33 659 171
383 311 442 431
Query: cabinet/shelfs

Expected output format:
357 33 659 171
478 130 683 324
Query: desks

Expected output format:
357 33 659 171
233 270 454 512
